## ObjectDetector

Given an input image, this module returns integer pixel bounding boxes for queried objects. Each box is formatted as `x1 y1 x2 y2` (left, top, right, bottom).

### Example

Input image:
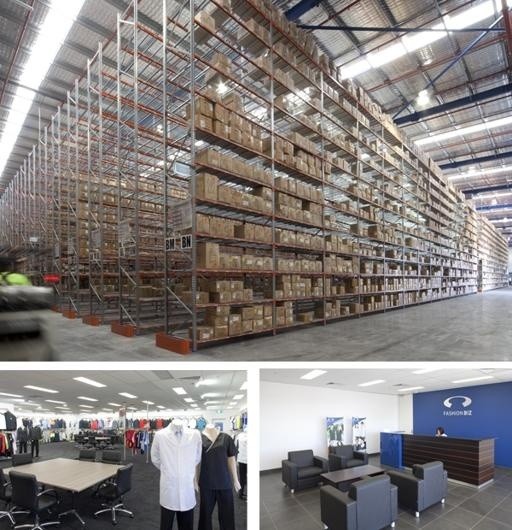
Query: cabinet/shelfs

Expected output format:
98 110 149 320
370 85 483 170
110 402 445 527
1 0 508 354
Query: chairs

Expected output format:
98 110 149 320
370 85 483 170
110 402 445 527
281 445 448 530
0 448 135 530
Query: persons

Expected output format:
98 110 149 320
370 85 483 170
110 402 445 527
151 419 202 530
233 428 247 501
199 424 242 530
435 426 447 436
329 420 367 449
1 254 32 286
16 424 29 453
29 424 42 457
